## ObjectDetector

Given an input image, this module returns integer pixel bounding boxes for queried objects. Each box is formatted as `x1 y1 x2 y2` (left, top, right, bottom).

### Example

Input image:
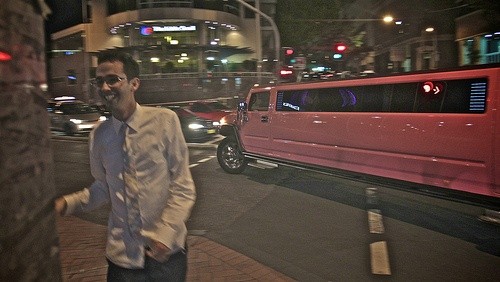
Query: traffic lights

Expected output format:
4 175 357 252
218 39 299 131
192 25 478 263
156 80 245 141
283 46 296 66
330 41 348 62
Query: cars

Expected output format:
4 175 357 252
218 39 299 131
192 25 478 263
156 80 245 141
216 66 500 209
161 100 232 142
47 96 108 136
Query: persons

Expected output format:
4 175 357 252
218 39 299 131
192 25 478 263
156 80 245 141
55 51 196 282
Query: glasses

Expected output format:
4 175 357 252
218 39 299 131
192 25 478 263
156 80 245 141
91 77 129 88
